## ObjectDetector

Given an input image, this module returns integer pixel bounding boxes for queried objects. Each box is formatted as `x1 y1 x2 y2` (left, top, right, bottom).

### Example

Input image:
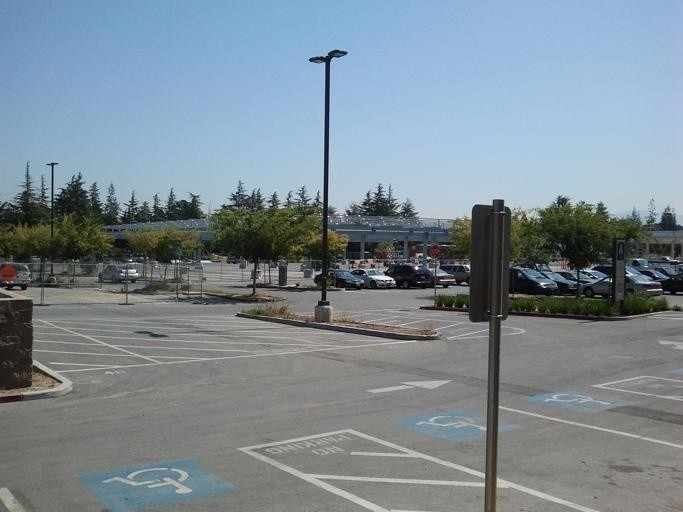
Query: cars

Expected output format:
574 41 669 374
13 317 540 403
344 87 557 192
314 269 365 290
429 268 457 288
509 256 683 298
98 264 139 283
351 268 397 289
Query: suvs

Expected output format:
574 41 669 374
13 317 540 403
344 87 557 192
382 264 435 289
0 262 32 291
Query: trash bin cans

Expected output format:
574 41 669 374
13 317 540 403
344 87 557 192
278 265 287 285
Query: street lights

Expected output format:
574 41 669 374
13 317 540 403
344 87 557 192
46 162 59 276
309 48 349 306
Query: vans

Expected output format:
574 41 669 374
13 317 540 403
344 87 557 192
440 263 470 286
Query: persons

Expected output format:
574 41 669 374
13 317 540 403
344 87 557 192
336 255 440 270
